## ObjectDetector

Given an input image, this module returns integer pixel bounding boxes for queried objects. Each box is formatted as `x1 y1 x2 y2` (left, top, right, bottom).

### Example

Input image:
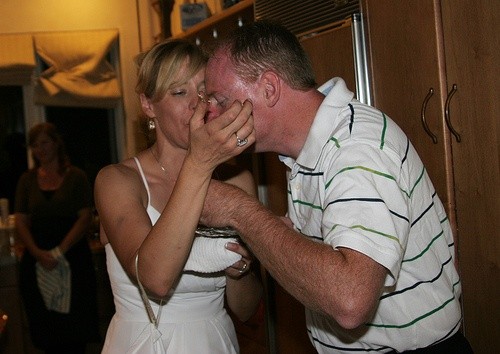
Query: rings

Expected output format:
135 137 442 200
235 131 247 147
239 263 246 274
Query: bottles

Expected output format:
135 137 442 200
5 215 23 257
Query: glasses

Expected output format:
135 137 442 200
31 139 51 148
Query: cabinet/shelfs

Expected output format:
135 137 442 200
365 0 500 354
264 15 365 354
135 0 275 354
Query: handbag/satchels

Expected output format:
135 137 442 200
121 249 166 354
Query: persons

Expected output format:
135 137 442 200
95 38 265 354
13 124 92 354
197 21 470 354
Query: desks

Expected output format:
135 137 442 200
0 236 116 354
